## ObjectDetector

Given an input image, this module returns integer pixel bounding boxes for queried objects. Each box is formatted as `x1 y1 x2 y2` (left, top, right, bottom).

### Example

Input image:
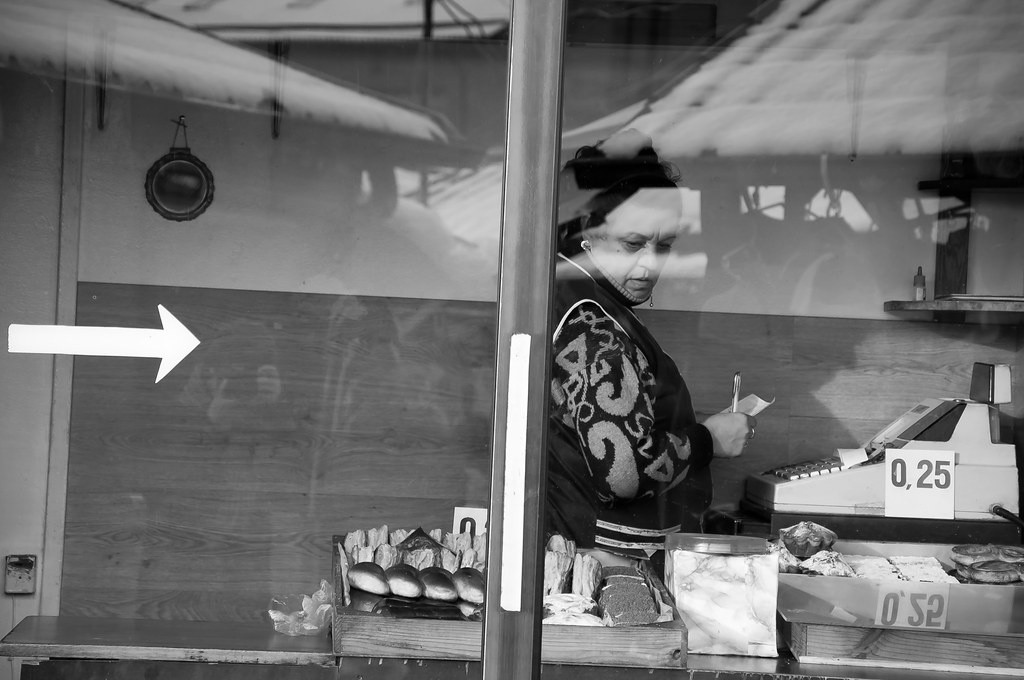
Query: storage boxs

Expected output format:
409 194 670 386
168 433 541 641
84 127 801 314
664 538 780 657
330 535 689 669
774 539 1024 676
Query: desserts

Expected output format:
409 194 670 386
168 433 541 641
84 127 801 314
777 521 854 576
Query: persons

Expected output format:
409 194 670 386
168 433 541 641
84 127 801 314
542 128 758 576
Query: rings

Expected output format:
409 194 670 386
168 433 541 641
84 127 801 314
744 442 749 448
749 428 755 439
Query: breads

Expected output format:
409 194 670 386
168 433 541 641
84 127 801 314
842 555 961 584
948 545 1024 586
341 523 663 627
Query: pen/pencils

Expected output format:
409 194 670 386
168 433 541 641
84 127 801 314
732 370 742 413
